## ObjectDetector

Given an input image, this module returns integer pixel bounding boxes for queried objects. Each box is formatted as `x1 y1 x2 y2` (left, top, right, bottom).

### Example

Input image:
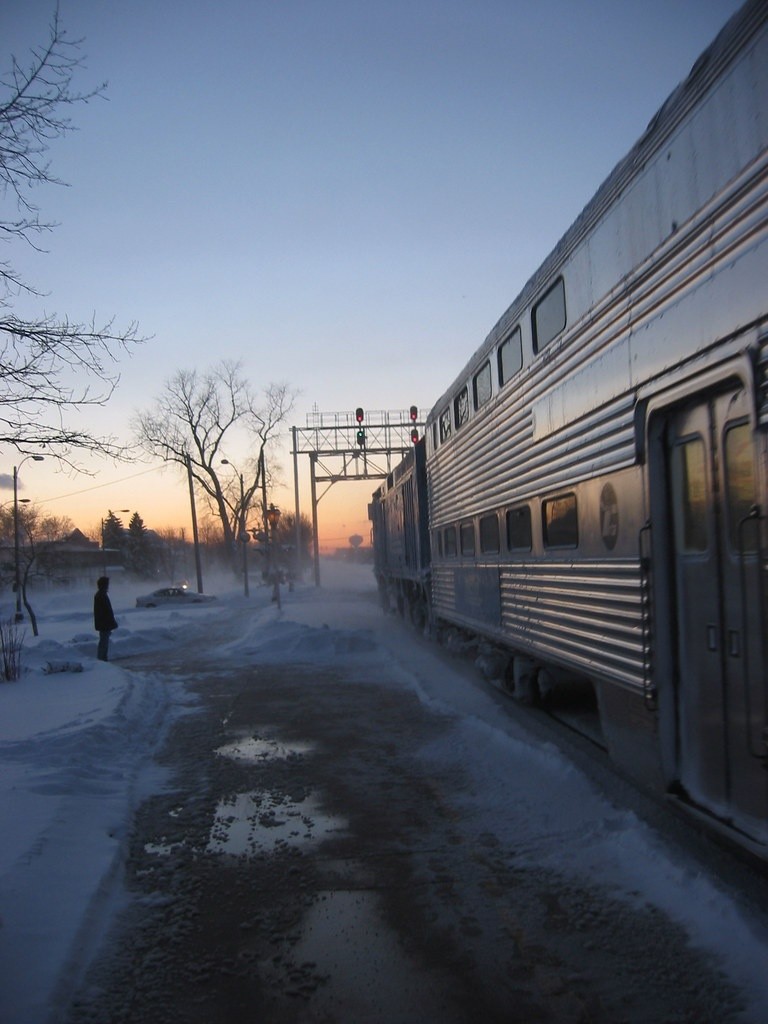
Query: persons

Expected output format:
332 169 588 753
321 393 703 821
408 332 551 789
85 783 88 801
93 576 118 661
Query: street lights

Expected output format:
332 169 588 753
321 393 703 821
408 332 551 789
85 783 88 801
102 509 130 579
0 499 31 510
221 459 251 597
266 503 283 610
12 456 45 621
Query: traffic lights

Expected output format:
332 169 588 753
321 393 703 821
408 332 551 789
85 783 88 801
411 430 419 443
410 405 418 420
357 431 365 444
356 408 364 422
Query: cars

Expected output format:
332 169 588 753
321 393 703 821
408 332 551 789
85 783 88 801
136 587 218 610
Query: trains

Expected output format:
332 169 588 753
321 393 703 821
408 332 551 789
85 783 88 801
367 0 768 857
